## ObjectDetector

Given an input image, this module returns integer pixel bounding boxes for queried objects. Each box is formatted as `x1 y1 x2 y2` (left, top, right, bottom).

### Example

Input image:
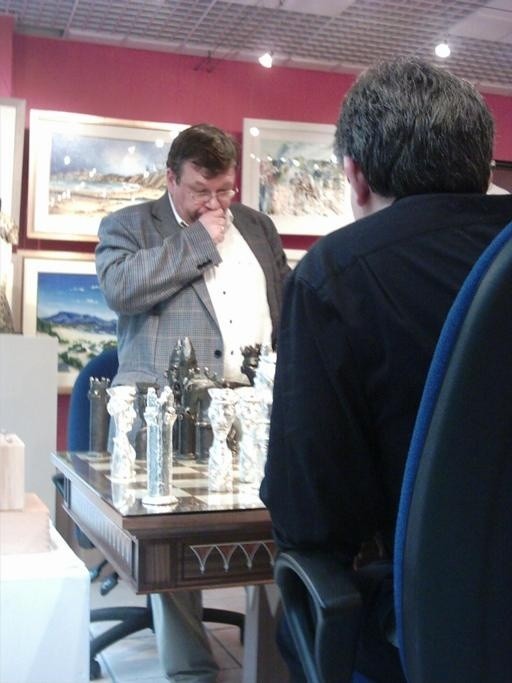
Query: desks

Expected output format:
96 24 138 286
52 449 292 683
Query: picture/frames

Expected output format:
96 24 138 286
1 250 23 333
25 108 193 244
1 93 26 249
238 116 354 237
280 247 309 272
23 245 118 398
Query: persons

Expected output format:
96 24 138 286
93 125 292 682
260 55 512 682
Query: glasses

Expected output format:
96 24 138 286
178 182 238 203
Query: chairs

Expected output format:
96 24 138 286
53 341 243 683
244 217 509 683
